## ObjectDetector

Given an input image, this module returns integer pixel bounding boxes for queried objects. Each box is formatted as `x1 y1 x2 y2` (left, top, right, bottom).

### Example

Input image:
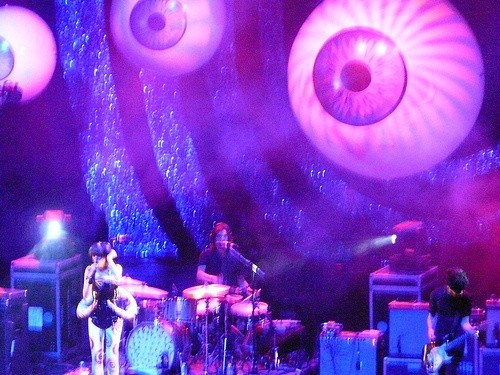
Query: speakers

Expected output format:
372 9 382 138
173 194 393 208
319 300 500 375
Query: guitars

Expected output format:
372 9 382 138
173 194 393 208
420 319 492 373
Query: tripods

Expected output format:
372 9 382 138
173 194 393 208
187 247 302 375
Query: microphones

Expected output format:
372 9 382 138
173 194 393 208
111 235 128 241
215 241 237 248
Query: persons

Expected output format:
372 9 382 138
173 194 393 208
196 222 260 300
83 241 130 375
426 267 479 375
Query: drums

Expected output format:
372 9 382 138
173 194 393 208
161 295 193 324
123 321 181 374
226 292 246 304
188 296 224 321
228 299 268 354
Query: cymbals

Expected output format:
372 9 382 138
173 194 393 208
180 282 231 301
122 285 169 299
121 275 148 286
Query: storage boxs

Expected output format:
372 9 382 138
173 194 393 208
368 265 440 328
9 255 84 362
